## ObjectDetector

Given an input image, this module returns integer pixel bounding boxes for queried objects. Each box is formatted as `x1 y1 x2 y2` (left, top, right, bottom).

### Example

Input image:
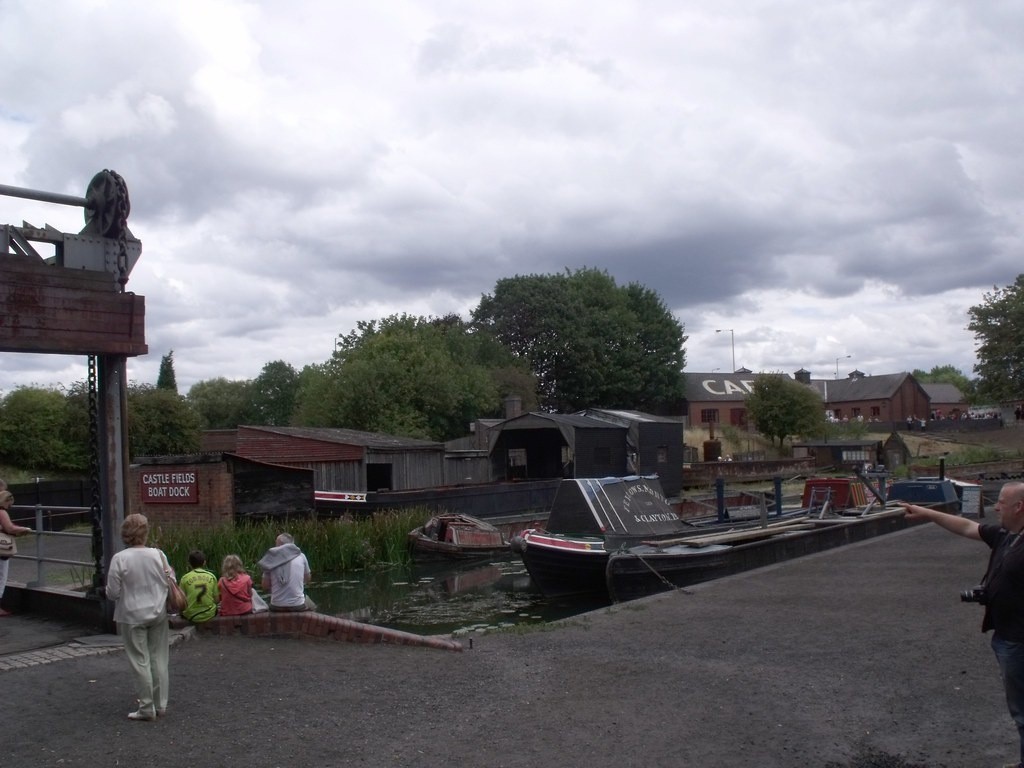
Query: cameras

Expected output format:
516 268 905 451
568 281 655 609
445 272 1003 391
960 585 986 605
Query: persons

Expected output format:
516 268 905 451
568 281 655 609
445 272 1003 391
1014 406 1021 419
262 533 312 611
896 482 1024 768
106 514 176 720
828 413 882 422
931 408 997 420
218 554 253 615
179 551 219 622
0 478 31 614
905 411 926 432
718 455 732 474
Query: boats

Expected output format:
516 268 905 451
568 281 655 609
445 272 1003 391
407 513 513 558
513 455 983 602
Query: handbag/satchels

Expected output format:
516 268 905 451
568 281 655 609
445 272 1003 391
0 532 16 558
156 548 189 615
251 588 270 614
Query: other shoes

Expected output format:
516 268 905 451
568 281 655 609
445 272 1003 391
0 609 11 616
128 710 156 719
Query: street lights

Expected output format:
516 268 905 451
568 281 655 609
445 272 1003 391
717 329 735 373
836 356 852 379
